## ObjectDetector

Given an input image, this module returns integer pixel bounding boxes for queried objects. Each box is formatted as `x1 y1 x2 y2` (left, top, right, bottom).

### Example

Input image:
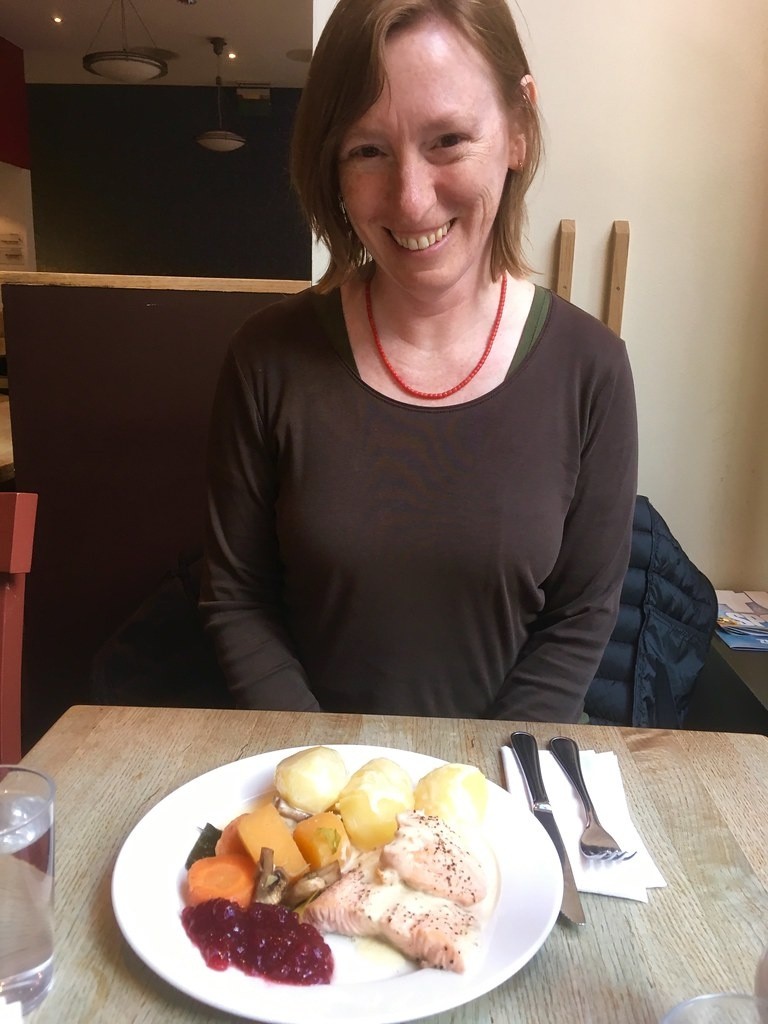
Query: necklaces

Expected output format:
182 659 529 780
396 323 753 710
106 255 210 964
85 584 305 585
363 270 507 400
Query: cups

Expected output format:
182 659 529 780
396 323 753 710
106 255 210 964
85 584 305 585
0 764 58 1024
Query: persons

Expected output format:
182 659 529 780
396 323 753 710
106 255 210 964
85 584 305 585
196 0 639 724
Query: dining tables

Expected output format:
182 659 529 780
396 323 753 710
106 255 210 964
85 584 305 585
0 704 767 1024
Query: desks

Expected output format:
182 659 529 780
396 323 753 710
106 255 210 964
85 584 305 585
691 591 768 737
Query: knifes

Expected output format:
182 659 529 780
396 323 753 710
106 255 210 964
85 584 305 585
509 730 588 925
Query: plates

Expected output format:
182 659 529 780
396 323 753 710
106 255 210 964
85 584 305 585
110 743 564 1024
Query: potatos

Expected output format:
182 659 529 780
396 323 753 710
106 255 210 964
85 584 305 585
414 763 486 825
338 758 410 847
275 748 347 813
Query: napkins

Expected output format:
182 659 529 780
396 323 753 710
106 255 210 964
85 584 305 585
502 745 667 904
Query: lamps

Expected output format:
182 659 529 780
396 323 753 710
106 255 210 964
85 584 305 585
82 0 168 85
194 38 247 153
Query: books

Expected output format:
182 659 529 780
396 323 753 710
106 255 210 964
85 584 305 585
712 590 768 650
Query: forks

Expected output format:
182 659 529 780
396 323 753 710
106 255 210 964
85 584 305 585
549 736 638 864
